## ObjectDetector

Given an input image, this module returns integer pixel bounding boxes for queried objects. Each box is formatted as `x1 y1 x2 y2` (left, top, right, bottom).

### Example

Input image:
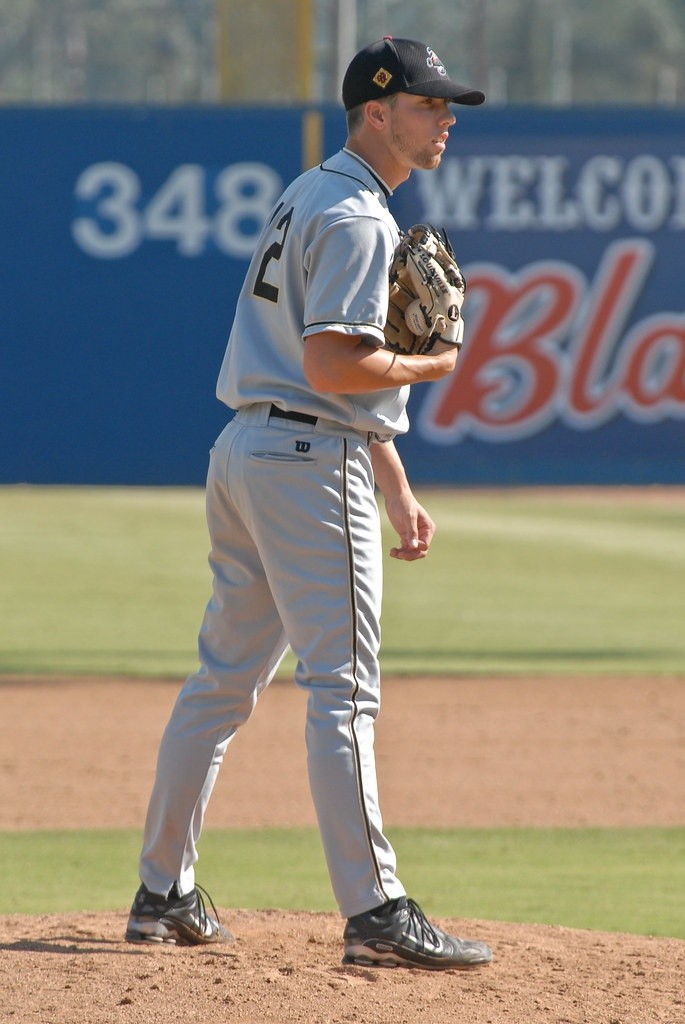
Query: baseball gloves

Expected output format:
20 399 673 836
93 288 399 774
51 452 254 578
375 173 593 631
380 220 472 363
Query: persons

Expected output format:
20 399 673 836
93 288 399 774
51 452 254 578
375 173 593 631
121 35 490 969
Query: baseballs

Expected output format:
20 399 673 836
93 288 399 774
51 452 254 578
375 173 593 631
403 296 436 339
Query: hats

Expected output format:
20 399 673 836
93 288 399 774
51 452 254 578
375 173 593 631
342 36 486 111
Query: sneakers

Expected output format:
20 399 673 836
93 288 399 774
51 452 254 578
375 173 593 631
125 881 237 947
342 896 491 970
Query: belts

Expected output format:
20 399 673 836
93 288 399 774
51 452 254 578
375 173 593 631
269 403 318 426
